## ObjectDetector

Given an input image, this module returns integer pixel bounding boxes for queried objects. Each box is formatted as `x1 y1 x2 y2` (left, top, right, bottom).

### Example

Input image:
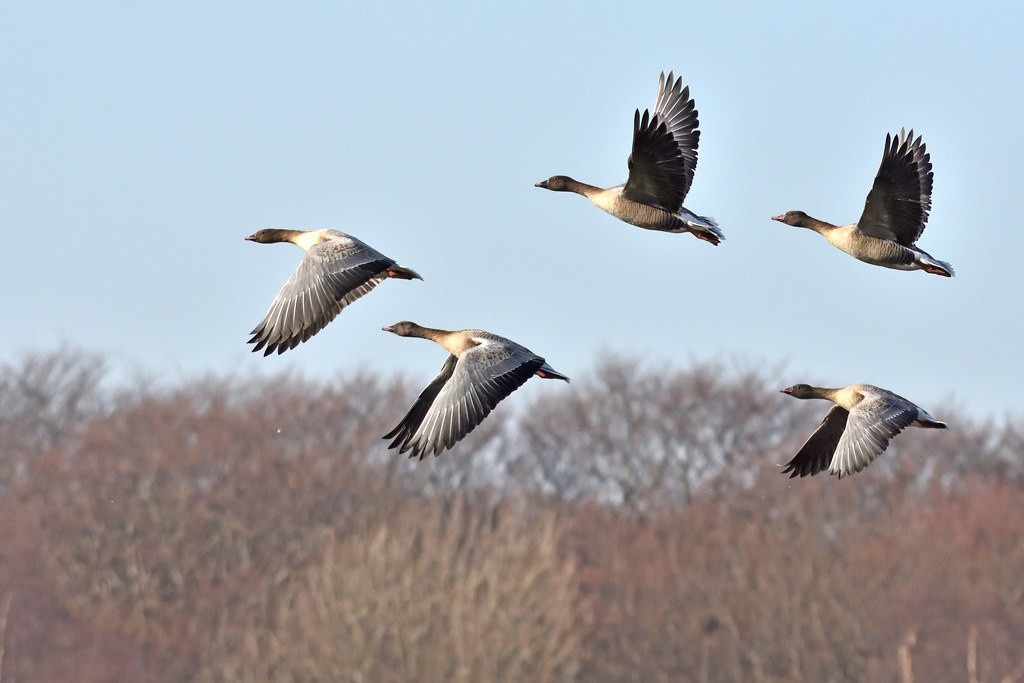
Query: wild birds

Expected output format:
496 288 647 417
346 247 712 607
779 383 949 480
381 320 571 461
533 70 728 247
771 126 957 279
243 228 425 357
